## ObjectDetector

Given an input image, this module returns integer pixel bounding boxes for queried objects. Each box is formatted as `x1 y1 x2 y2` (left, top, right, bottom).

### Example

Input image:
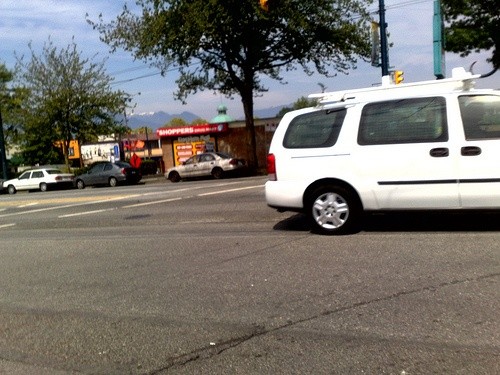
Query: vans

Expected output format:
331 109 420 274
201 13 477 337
263 67 500 234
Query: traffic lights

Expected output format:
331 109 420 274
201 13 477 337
395 70 404 84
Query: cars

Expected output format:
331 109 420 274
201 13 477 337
164 152 247 183
3 168 75 193
72 161 143 188
